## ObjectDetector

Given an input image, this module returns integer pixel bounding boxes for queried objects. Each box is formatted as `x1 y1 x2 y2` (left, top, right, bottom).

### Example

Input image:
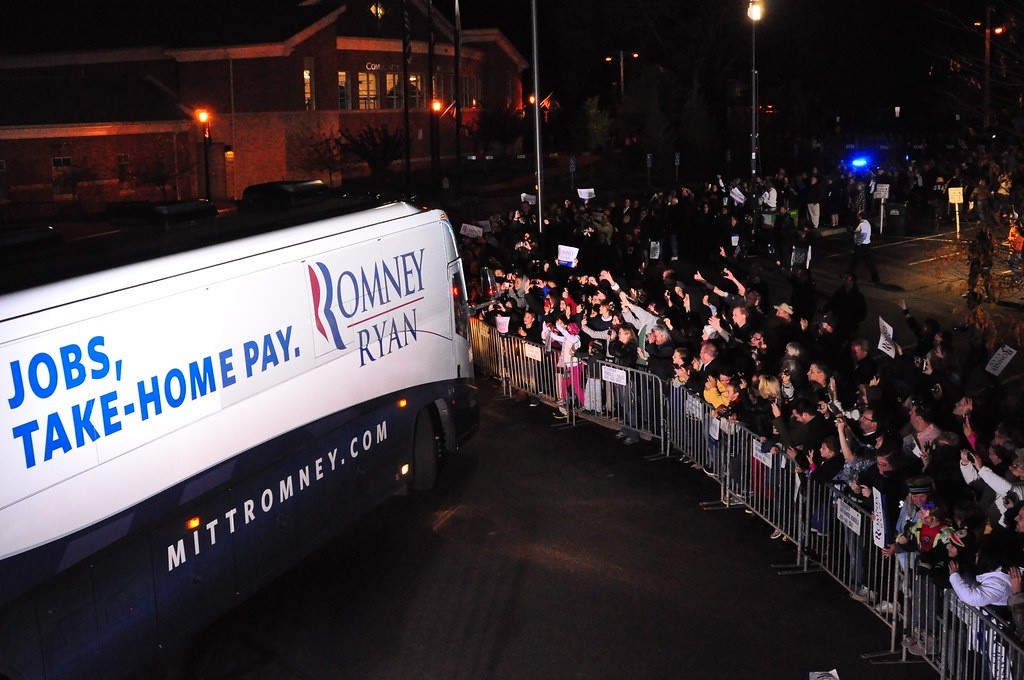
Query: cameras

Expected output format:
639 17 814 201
784 370 790 376
932 385 939 393
834 418 841 424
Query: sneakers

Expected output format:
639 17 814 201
722 495 745 505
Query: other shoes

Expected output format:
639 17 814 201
902 633 939 655
782 536 789 541
770 529 781 539
962 292 967 297
552 410 561 415
983 296 995 303
554 412 567 418
623 437 640 445
850 585 877 602
615 427 631 438
875 601 900 613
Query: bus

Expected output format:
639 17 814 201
0 192 500 680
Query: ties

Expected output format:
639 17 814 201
700 364 705 371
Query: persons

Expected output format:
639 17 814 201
436 138 1022 679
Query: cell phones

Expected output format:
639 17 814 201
923 361 926 370
774 397 779 406
856 482 863 489
967 453 975 464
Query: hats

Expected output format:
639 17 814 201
908 474 936 492
950 528 976 554
567 322 581 336
774 303 793 314
588 339 604 351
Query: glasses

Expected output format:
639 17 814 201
750 335 760 340
861 415 873 422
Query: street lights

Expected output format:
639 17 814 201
746 3 763 178
983 25 1007 128
604 48 640 93
199 111 211 199
